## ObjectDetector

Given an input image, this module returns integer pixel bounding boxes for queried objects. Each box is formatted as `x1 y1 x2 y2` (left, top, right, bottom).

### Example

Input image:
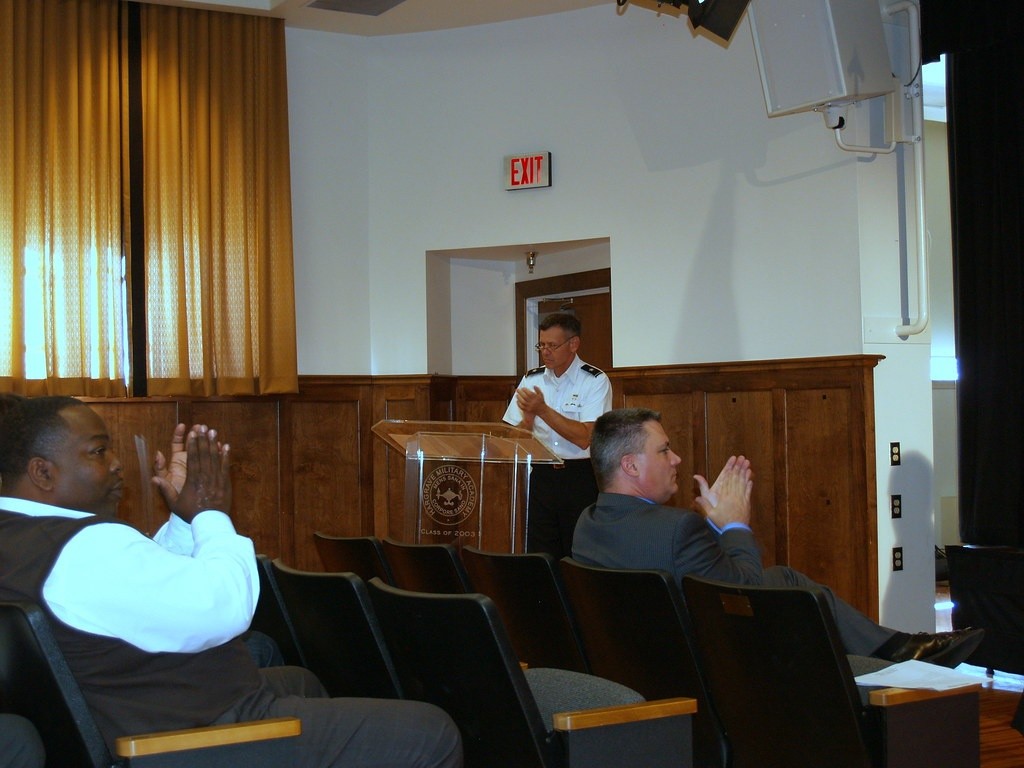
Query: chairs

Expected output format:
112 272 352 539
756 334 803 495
6 533 1022 768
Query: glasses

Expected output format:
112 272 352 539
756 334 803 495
534 334 576 353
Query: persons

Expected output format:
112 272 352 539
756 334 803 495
571 407 985 669
0 394 464 768
501 312 612 557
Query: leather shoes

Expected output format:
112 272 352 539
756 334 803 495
891 627 985 672
